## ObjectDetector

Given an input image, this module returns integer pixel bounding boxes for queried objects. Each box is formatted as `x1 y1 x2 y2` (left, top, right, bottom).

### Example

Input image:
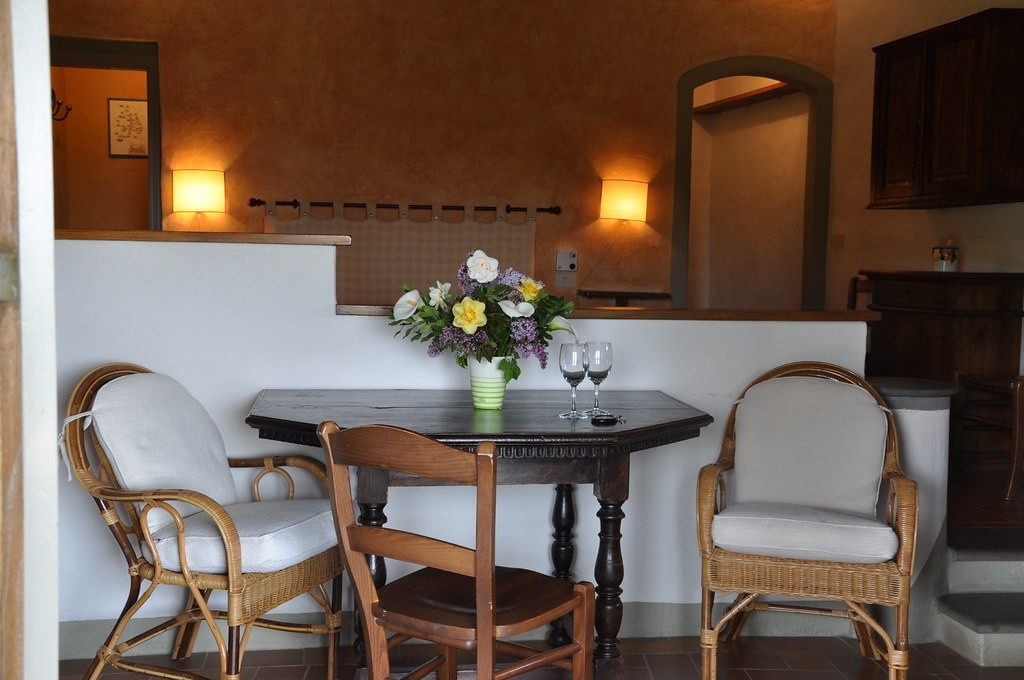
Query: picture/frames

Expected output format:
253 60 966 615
107 97 149 158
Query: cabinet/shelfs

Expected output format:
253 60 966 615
865 8 1024 210
851 268 1023 471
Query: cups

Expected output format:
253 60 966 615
932 245 960 272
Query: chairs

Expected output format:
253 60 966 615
58 362 348 679
696 361 919 680
950 318 1024 502
317 419 595 680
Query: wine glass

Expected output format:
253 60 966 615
582 341 614 415
559 343 591 419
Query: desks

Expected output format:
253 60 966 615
245 389 714 659
579 290 671 307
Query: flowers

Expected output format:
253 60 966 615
389 249 580 384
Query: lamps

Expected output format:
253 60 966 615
171 169 226 212
600 178 647 223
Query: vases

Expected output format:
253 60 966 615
465 355 511 409
